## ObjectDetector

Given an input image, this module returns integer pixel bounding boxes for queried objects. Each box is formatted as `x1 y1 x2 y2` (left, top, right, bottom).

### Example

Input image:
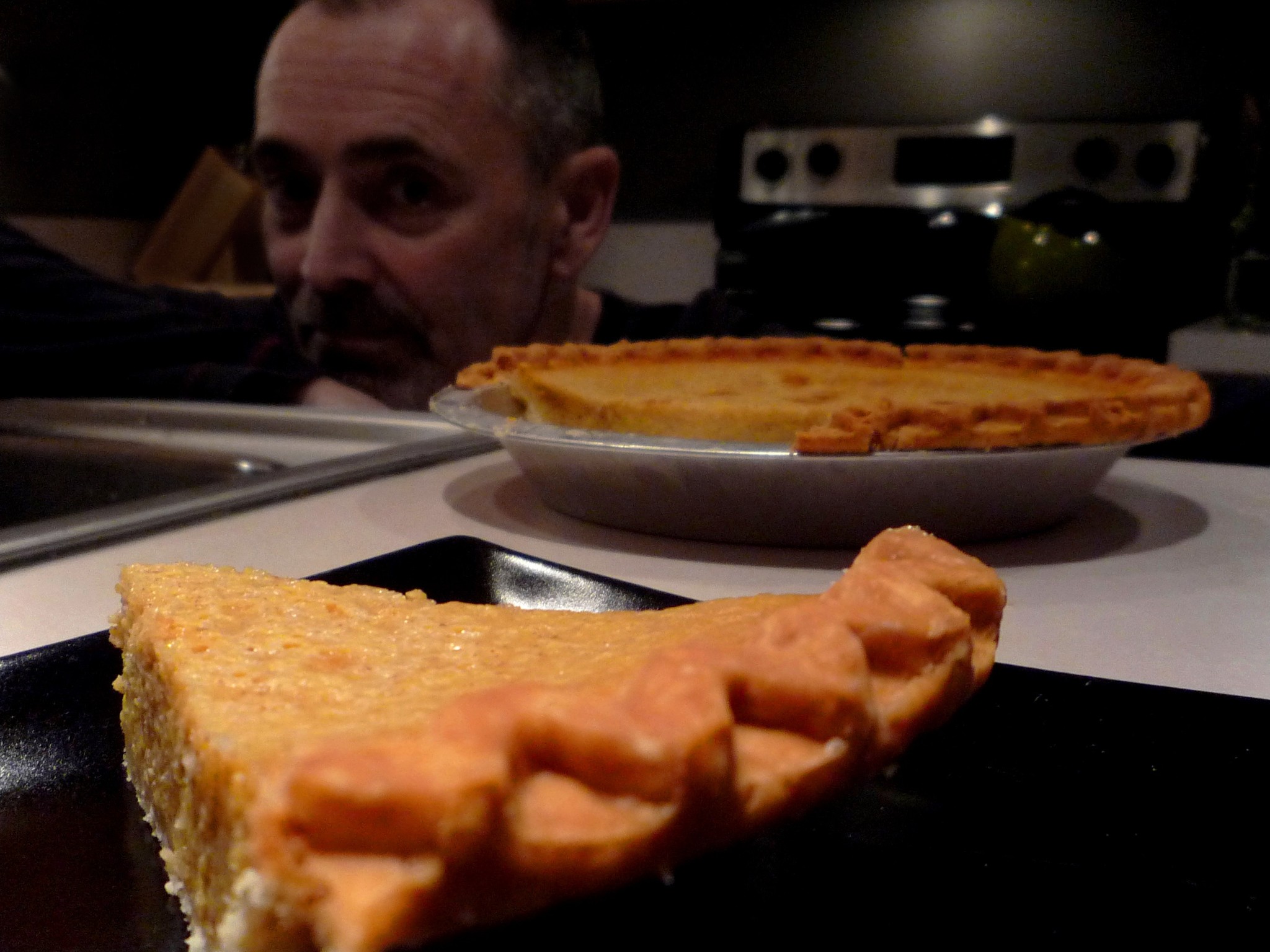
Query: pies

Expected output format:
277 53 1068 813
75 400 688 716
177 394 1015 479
456 335 1210 455
108 523 1014 952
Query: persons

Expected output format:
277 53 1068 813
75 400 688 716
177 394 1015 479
239 1 693 415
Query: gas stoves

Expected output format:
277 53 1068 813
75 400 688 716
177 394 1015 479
695 119 1212 358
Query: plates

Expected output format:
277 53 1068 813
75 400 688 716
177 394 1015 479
0 532 1270 952
425 387 1189 549
